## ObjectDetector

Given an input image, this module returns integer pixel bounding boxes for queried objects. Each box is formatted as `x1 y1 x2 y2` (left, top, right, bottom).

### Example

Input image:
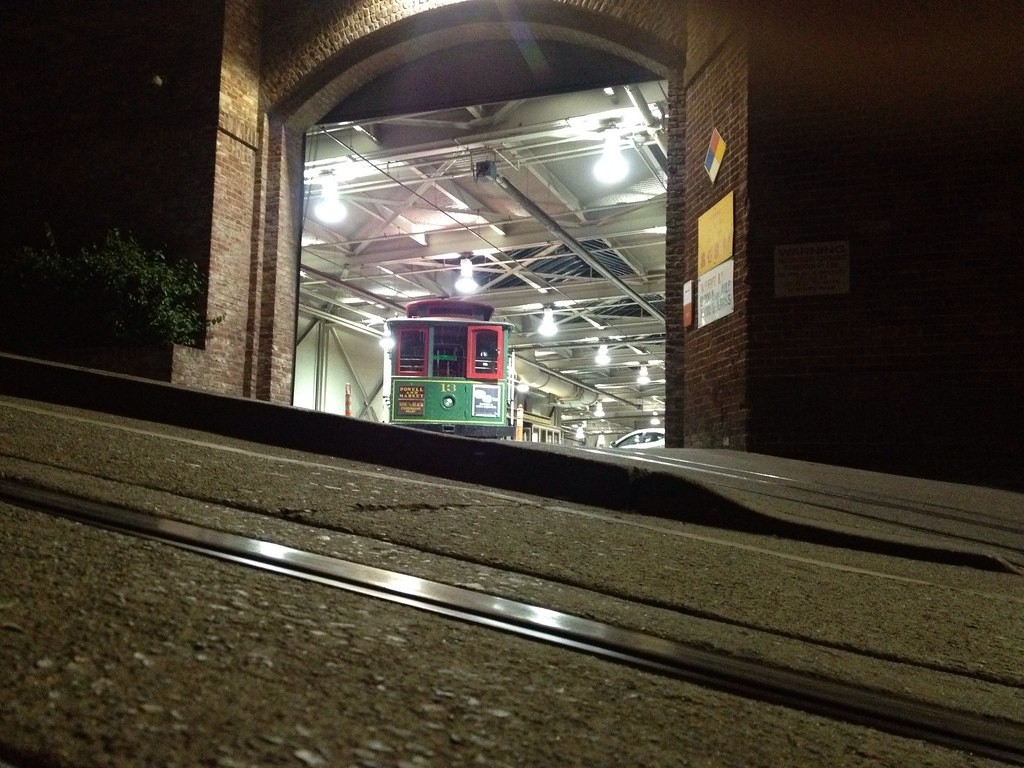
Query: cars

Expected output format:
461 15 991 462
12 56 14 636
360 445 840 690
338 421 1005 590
609 428 665 450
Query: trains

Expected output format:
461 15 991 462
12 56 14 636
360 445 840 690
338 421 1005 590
381 300 516 439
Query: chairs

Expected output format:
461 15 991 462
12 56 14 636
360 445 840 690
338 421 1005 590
650 435 658 442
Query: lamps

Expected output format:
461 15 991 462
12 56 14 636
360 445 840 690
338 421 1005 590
455 251 478 293
595 338 612 366
470 148 498 183
537 305 559 338
379 321 396 349
517 380 529 393
576 424 585 438
313 170 348 225
597 431 606 444
593 399 605 417
637 361 651 385
594 117 630 184
651 411 661 425
